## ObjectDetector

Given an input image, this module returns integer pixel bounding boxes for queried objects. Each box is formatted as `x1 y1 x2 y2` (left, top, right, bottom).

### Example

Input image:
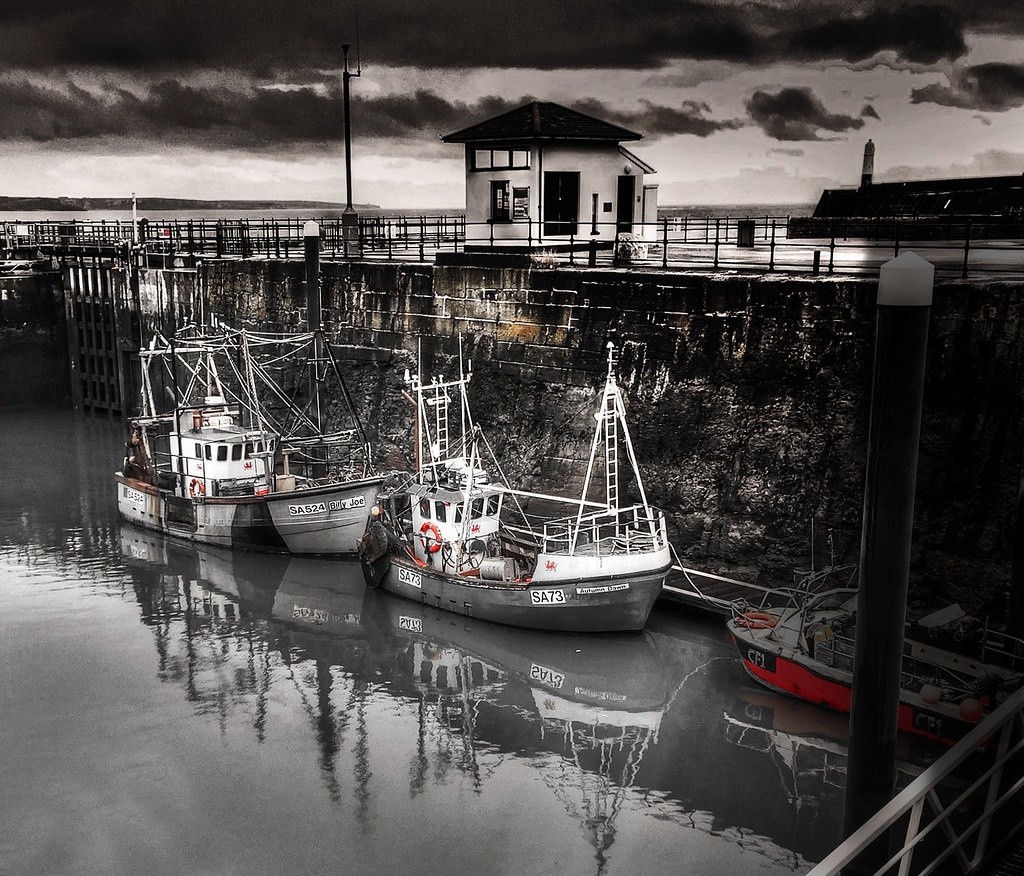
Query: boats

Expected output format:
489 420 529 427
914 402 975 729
724 517 1024 758
722 682 983 852
354 330 676 638
113 311 397 559
116 522 367 804
354 587 672 876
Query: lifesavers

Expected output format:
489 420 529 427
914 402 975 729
736 612 777 629
419 522 443 553
189 478 205 498
